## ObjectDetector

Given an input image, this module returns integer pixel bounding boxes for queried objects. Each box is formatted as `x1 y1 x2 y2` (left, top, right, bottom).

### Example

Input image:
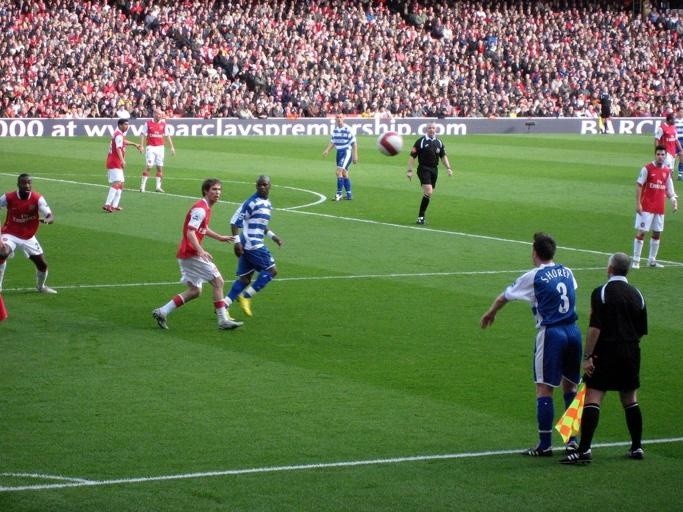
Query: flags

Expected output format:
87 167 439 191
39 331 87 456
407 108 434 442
555 381 586 445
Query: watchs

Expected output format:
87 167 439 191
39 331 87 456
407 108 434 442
583 352 593 359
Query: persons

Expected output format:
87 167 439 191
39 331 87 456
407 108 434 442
655 113 682 175
479 232 582 457
0 232 8 320
631 146 678 268
322 112 358 202
1 1 683 119
557 252 648 461
101 119 143 213
0 174 58 294
139 108 175 192
673 107 683 180
223 176 283 316
407 122 453 225
152 179 244 331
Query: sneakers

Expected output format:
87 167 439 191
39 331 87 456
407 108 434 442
557 449 592 464
37 286 57 295
520 445 553 457
565 441 578 454
416 217 425 224
632 260 640 269
214 295 253 318
629 447 644 460
218 320 243 330
647 261 664 267
151 308 169 330
600 129 607 134
678 172 683 180
140 187 164 193
332 193 352 201
102 204 122 212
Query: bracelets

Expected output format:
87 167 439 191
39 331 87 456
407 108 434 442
407 170 413 172
266 230 275 239
232 235 241 244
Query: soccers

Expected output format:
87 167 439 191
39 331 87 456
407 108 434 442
376 131 402 156
